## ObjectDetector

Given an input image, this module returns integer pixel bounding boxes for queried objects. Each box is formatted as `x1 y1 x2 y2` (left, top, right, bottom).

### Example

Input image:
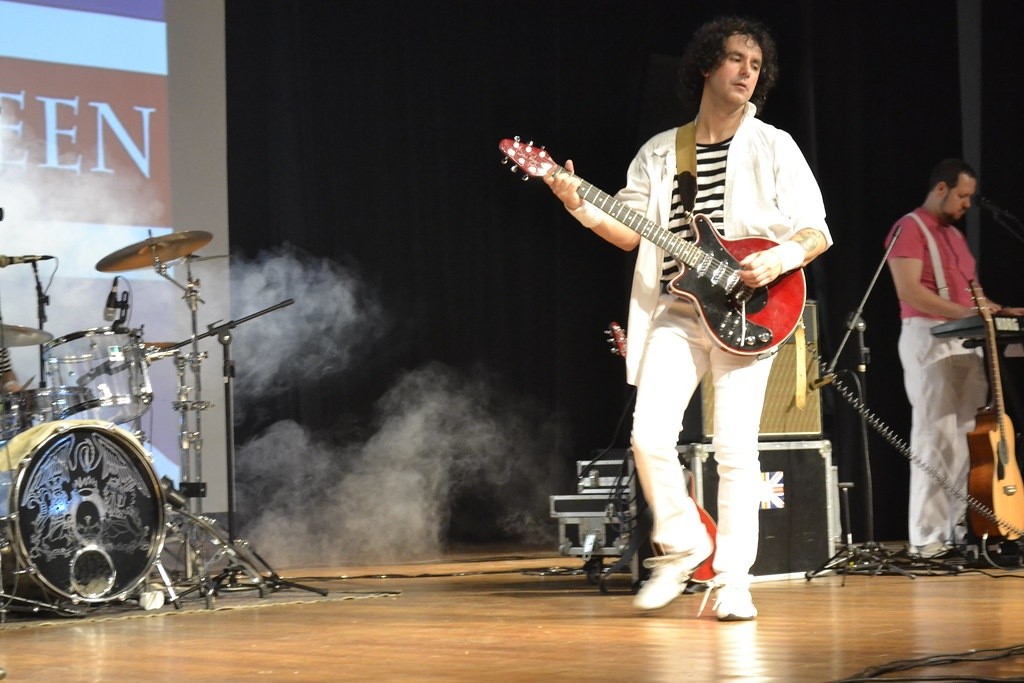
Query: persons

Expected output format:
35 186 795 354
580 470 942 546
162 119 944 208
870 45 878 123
884 161 1024 560
0 314 24 393
544 18 834 620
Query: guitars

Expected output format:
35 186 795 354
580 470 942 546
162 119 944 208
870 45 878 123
962 276 1023 544
497 134 808 361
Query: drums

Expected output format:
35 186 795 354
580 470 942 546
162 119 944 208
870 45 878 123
0 385 95 442
1 419 169 608
42 325 153 426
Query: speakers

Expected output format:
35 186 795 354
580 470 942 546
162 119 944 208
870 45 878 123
698 303 823 441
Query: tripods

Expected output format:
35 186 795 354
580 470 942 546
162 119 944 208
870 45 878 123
111 254 330 612
804 221 965 588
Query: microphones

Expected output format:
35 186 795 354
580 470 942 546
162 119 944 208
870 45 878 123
809 370 850 391
0 255 52 268
76 358 118 387
104 277 119 321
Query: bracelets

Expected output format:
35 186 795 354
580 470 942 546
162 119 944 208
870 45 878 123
564 200 604 228
998 307 1005 317
772 241 804 273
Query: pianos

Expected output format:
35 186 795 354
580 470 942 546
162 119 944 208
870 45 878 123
928 313 1024 340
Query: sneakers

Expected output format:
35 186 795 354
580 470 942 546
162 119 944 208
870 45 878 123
696 577 757 620
632 534 712 610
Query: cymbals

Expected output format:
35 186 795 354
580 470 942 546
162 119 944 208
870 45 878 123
95 229 215 275
0 324 54 348
143 341 181 361
